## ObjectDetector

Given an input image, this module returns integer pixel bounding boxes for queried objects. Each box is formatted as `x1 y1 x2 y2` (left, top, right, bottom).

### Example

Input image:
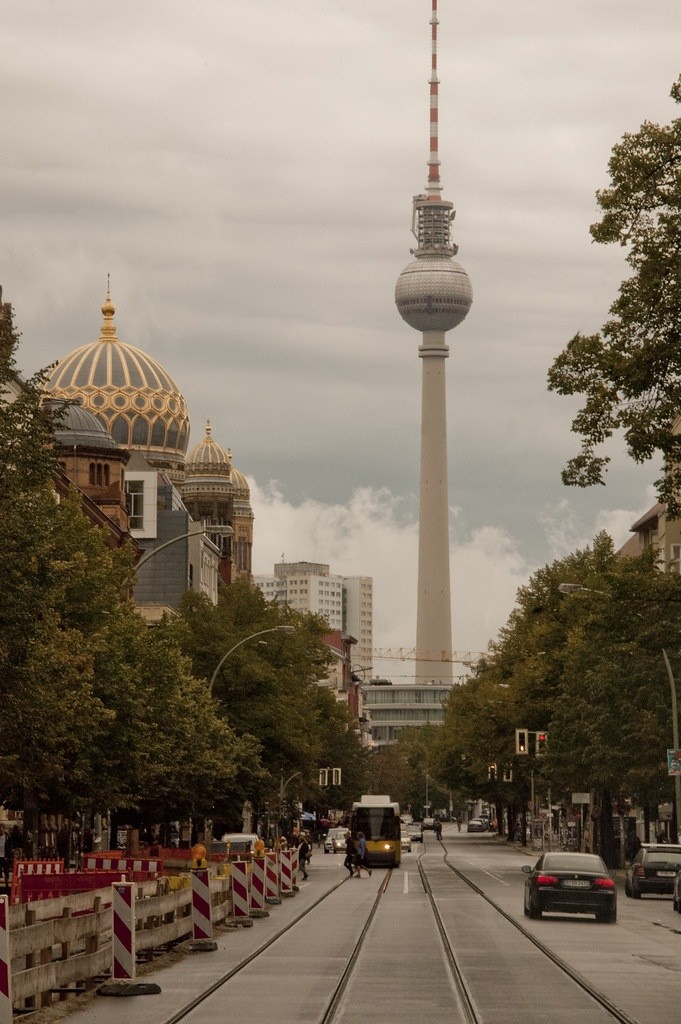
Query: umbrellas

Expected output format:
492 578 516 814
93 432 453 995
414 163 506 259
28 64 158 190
299 811 316 825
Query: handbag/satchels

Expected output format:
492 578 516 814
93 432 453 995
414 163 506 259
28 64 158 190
304 852 313 859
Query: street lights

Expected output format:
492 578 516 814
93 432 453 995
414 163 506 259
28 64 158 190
118 525 238 602
557 582 680 839
209 626 297 692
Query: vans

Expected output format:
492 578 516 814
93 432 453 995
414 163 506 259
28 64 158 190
220 831 260 853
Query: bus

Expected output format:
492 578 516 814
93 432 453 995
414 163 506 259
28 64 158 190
348 794 405 867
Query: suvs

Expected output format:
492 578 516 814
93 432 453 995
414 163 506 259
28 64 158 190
323 827 349 854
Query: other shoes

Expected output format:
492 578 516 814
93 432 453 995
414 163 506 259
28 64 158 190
302 874 309 880
350 871 354 877
369 869 372 876
354 874 361 878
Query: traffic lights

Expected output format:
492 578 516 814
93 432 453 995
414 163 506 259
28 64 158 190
535 731 549 759
503 763 513 782
488 762 496 781
516 728 528 754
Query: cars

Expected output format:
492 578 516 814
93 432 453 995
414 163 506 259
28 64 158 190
671 867 681 913
624 842 681 898
400 813 424 852
437 813 457 823
520 852 617 922
422 818 435 831
332 831 352 853
467 814 490 833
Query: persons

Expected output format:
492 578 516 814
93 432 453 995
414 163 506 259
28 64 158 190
457 817 462 832
0 823 25 879
626 832 641 860
320 817 330 840
56 823 74 867
433 820 442 840
281 832 313 880
343 831 371 878
668 757 681 774
